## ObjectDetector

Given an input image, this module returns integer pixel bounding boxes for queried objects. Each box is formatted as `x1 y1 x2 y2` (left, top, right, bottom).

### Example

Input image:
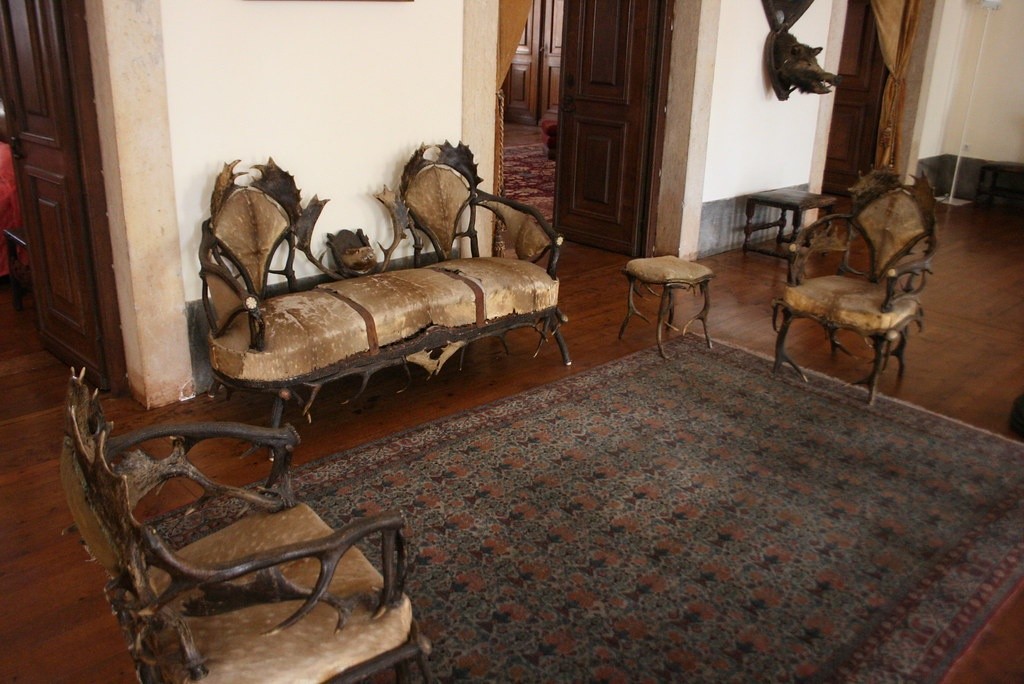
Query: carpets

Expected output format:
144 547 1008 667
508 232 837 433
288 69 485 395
88 332 1024 684
502 140 556 237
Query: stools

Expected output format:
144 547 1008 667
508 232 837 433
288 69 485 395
617 256 714 360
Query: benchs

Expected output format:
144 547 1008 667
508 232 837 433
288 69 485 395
200 140 571 461
64 372 436 684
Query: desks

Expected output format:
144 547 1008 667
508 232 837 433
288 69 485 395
4 226 38 331
743 184 836 261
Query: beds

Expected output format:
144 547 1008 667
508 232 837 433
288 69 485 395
0 141 29 277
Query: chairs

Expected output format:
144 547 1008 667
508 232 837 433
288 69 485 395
772 171 936 405
